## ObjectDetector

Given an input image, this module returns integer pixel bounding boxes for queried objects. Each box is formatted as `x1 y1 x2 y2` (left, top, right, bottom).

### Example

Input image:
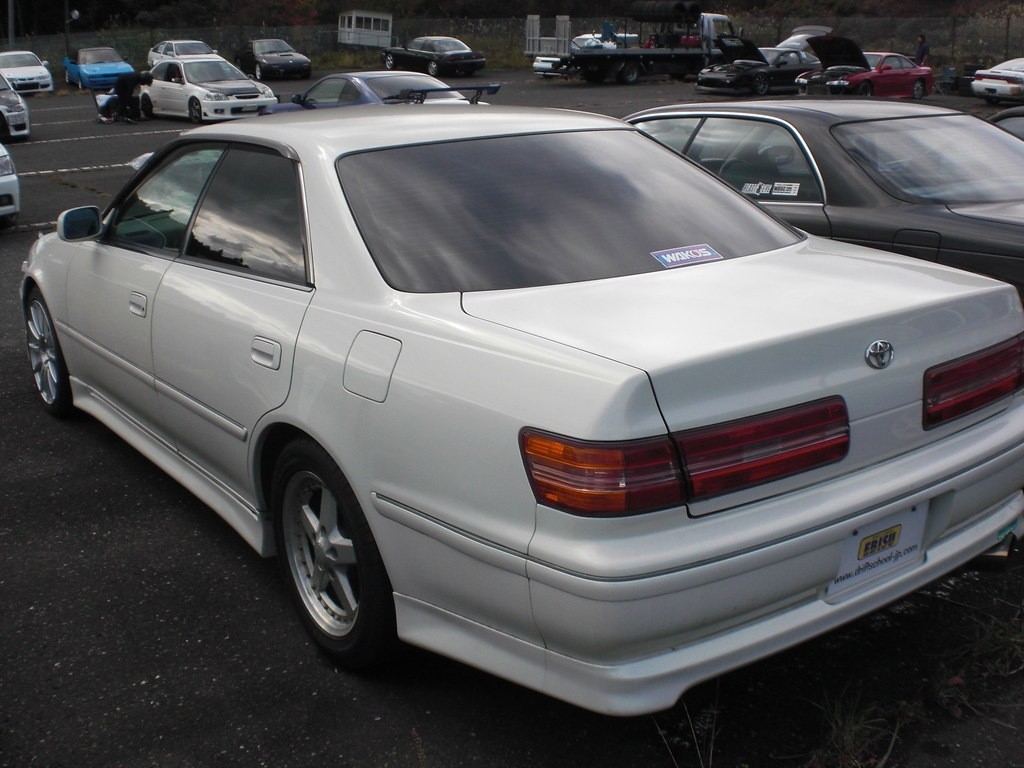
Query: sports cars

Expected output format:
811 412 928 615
257 71 501 119
63 46 135 90
695 24 933 100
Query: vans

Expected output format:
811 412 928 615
572 33 639 49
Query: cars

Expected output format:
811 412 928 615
138 40 278 123
19 99 1024 718
971 57 1024 103
381 36 486 77
235 39 311 81
0 50 54 227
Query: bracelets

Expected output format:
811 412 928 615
922 63 924 64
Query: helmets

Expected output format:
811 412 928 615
140 71 153 87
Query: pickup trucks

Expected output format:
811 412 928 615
533 42 616 79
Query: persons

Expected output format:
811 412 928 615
97 72 153 124
915 35 929 66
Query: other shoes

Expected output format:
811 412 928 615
137 116 148 121
124 117 139 124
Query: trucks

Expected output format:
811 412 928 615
570 12 744 85
